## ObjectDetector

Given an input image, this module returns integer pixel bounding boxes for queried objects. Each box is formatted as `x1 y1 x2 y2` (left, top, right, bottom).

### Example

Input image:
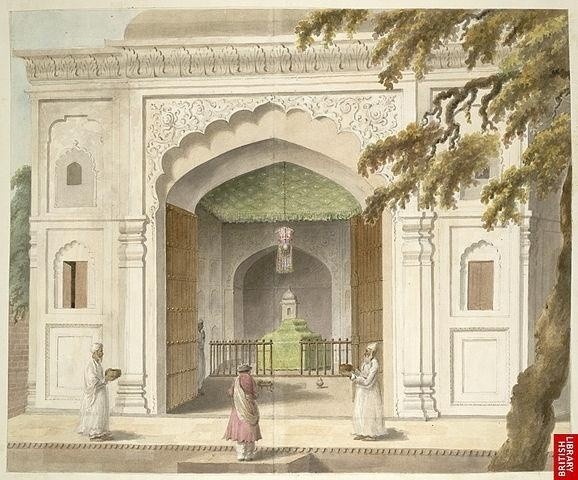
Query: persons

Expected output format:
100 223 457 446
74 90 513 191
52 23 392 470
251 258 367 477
77 343 117 442
197 319 209 394
339 340 389 440
221 365 263 462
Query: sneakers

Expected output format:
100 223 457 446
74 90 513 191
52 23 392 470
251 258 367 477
238 458 253 461
90 434 114 442
353 435 377 441
